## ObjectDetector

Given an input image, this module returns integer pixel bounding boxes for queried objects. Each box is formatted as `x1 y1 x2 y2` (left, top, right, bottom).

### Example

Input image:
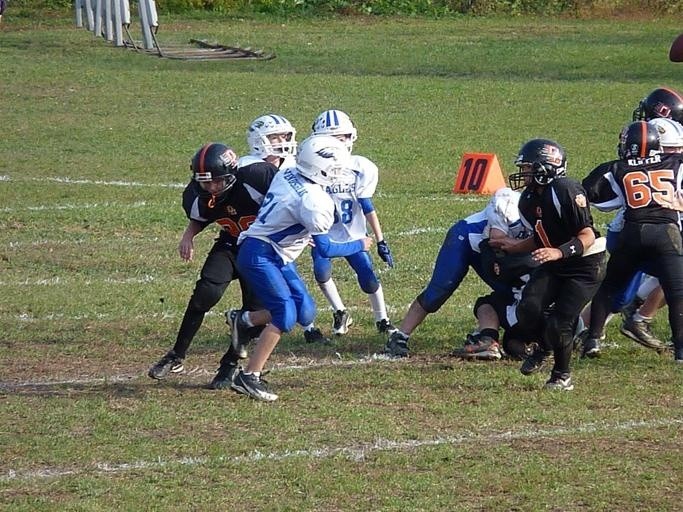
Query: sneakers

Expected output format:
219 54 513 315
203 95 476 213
211 363 278 401
225 310 249 358
454 335 501 359
375 318 410 357
521 299 665 390
149 351 184 379
304 310 352 342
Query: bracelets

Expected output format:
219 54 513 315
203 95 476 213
561 239 584 259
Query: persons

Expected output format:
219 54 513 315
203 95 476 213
312 110 400 338
450 227 538 360
226 115 331 344
224 135 372 401
148 143 281 381
381 185 534 358
669 34 682 62
569 118 683 339
581 121 683 361
620 87 683 323
491 138 606 391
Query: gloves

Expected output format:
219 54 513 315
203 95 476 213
377 241 393 267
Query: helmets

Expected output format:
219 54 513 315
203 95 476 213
510 139 567 190
191 143 237 208
618 87 683 159
248 110 357 186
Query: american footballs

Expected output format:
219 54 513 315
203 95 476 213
503 323 526 360
